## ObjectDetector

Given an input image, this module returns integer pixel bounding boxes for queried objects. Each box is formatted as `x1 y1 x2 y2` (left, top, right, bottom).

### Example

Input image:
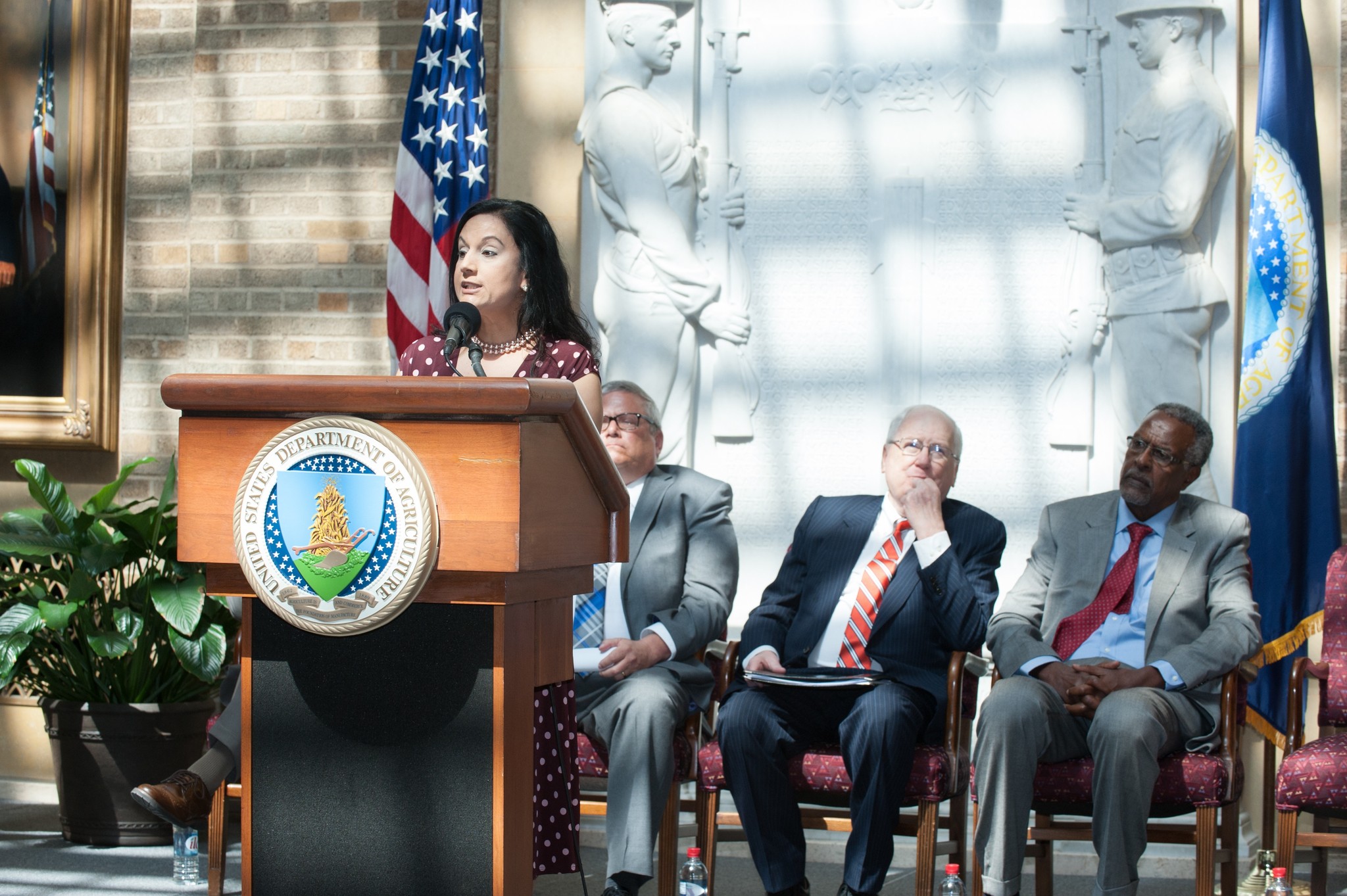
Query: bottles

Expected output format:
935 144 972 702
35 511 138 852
172 823 199 886
1265 867 1293 896
937 863 966 896
679 847 708 896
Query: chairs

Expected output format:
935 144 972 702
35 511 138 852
576 549 1347 896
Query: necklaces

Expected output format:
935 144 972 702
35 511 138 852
470 324 541 355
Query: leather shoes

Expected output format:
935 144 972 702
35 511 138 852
129 767 211 828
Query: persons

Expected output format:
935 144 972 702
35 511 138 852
1064 0 1236 503
128 599 243 831
715 406 1006 896
391 198 603 879
572 1 751 470
970 400 1263 896
572 379 740 895
0 162 19 290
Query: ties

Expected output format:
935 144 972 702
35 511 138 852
570 563 609 650
835 520 913 669
1048 524 1153 660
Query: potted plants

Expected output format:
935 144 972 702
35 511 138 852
0 454 234 845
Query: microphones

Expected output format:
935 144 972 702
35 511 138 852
443 302 481 357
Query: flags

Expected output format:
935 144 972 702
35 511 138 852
17 0 58 280
385 0 489 364
1225 0 1346 748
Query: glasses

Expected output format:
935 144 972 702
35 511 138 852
1125 434 1192 467
599 412 660 432
886 437 960 464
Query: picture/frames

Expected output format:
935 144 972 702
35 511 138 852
0 0 130 457
